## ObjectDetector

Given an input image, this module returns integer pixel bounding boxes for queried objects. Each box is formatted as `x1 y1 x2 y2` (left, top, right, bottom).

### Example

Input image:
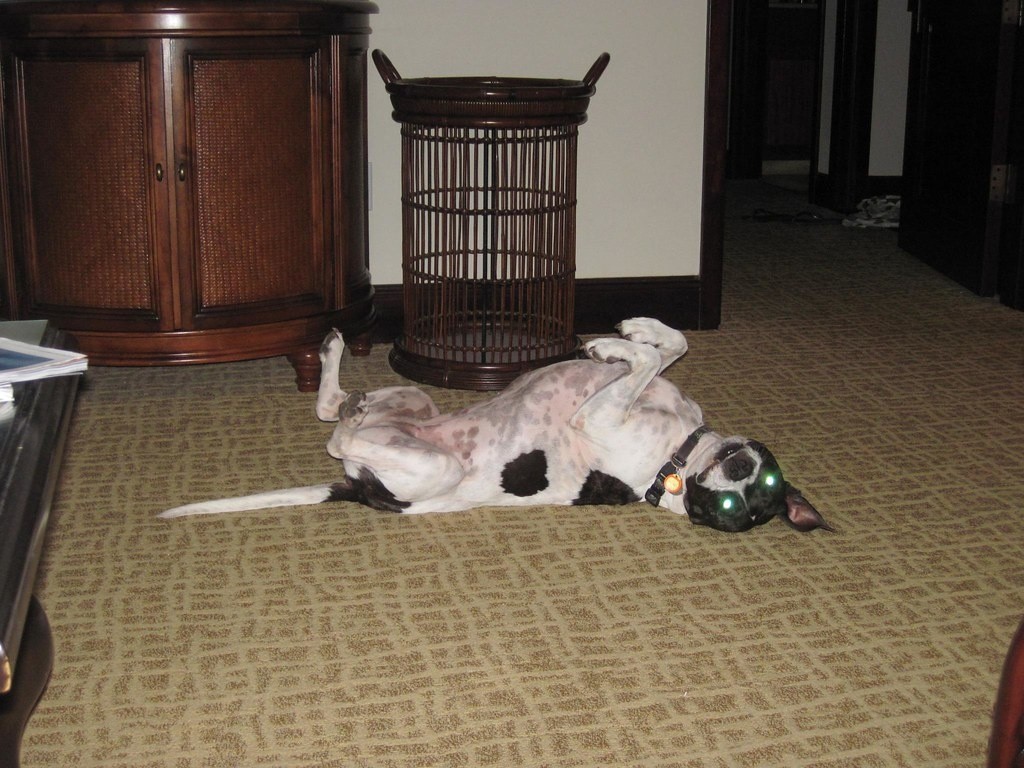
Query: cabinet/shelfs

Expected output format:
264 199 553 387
1 0 383 391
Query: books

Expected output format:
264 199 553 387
0 336 89 403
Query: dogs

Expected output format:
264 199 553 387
154 317 837 533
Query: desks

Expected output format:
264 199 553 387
1 320 88 767
